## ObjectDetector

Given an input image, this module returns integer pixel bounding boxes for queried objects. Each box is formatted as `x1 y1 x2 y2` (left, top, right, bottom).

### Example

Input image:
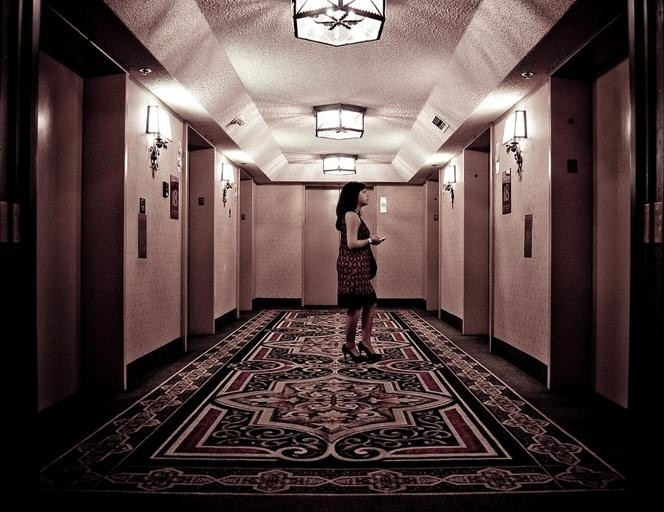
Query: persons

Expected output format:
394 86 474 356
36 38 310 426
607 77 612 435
336 181 387 363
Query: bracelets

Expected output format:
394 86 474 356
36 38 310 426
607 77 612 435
367 237 372 245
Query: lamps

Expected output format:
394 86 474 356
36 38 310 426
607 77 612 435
501 110 526 180
319 154 359 176
290 0 386 48
144 105 173 178
311 104 366 141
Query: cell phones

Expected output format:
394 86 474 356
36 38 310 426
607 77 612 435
379 237 386 241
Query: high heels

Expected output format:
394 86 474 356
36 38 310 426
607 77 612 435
341 341 383 362
